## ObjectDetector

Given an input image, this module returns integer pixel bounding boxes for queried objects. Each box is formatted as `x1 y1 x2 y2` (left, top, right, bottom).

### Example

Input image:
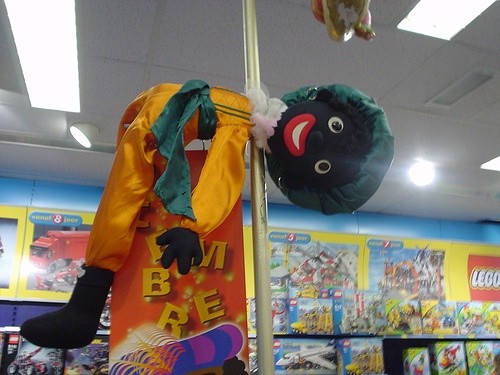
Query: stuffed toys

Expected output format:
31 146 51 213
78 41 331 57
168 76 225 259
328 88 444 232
20 80 393 349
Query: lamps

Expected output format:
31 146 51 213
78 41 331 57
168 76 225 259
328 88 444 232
70 122 101 148
